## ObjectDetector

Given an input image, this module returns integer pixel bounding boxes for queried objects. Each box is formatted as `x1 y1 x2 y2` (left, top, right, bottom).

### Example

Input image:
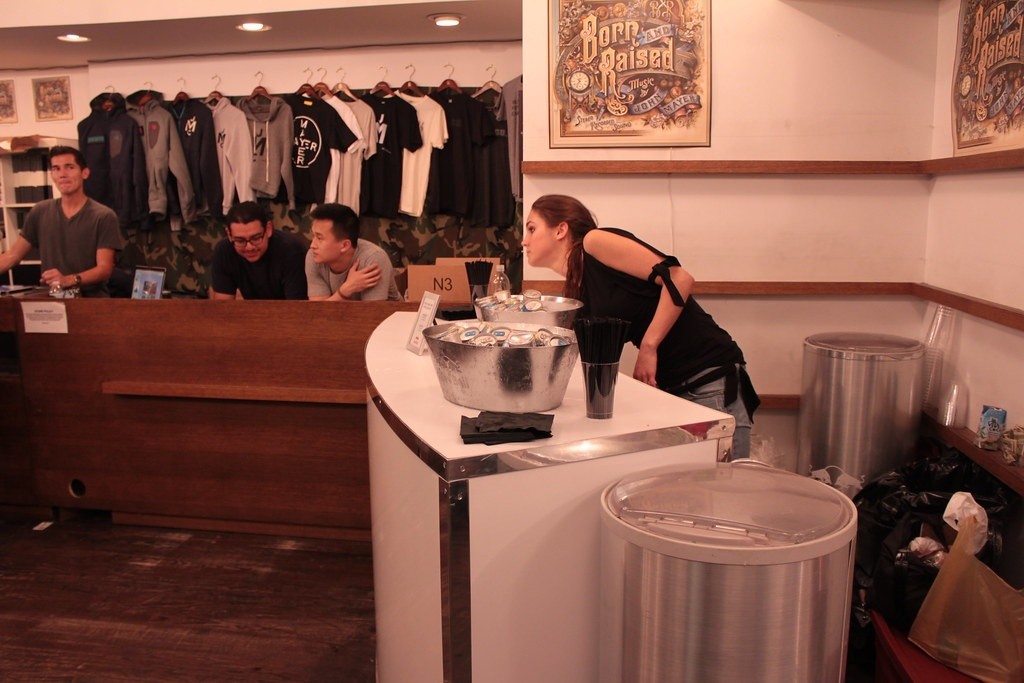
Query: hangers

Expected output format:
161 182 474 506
99 85 116 111
244 72 274 103
171 78 188 106
138 81 154 104
201 77 223 104
368 64 502 101
292 66 359 103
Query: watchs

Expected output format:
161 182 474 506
73 273 81 285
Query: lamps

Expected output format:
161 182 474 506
429 14 464 27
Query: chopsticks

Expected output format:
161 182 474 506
571 316 632 363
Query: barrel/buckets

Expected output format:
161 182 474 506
797 328 919 494
599 457 858 683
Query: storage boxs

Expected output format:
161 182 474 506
859 515 1024 683
407 258 500 303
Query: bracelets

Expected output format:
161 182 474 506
338 289 350 298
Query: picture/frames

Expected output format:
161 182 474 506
0 79 19 124
547 0 711 149
31 77 73 123
951 0 1024 157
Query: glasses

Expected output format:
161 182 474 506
232 222 269 247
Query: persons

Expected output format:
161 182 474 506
207 201 309 301
304 202 404 301
0 146 123 291
521 194 761 462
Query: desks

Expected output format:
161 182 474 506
922 406 1024 498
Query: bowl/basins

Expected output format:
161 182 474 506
473 295 585 329
422 322 580 414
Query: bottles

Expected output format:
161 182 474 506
50 280 64 298
490 265 511 298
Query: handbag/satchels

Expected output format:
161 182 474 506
851 469 1024 683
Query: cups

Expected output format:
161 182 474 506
581 363 619 420
920 303 967 428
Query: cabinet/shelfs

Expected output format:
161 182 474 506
0 146 62 290
